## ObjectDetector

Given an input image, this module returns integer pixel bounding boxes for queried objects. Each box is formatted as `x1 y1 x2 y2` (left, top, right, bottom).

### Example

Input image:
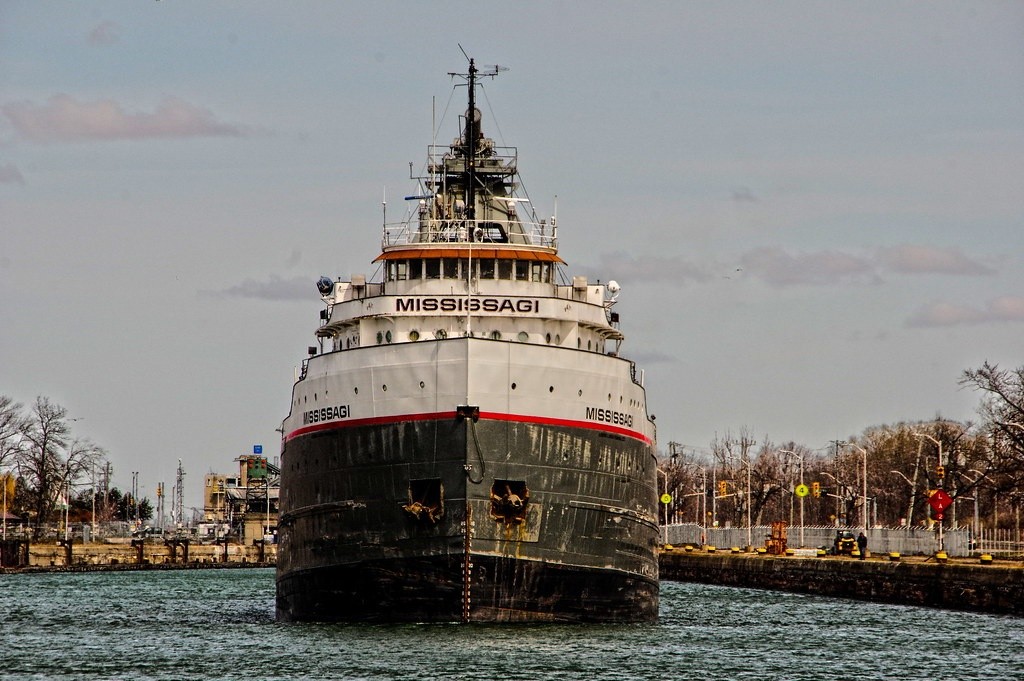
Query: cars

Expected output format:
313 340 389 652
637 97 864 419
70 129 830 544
133 526 169 538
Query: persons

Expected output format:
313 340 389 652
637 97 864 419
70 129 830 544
857 532 867 560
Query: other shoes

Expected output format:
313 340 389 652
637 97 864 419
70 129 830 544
860 557 866 560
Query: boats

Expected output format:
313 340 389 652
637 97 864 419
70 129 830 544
273 43 661 627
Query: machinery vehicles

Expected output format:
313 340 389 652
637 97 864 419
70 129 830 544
764 520 788 555
830 529 871 559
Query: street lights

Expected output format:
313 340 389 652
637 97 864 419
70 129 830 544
685 462 707 544
780 449 804 549
656 468 668 544
728 456 751 546
967 469 997 529
819 472 844 529
838 443 867 539
890 470 916 531
913 432 943 551
